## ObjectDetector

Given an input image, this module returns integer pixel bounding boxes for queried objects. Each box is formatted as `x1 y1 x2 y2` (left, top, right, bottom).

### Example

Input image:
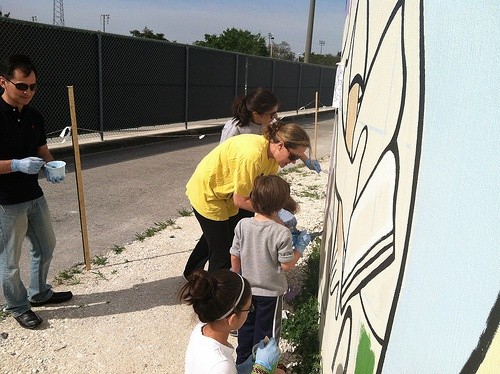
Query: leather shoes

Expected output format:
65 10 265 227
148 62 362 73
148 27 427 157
29 291 73 307
13 310 43 329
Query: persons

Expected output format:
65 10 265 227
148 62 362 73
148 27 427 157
182 122 312 282
229 174 311 365
0 53 73 329
176 268 281 374
219 87 321 263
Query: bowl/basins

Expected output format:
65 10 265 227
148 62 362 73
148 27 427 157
43 160 67 181
251 343 259 362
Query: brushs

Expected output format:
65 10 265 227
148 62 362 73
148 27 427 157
37 160 56 169
260 337 272 347
313 165 331 174
292 231 324 237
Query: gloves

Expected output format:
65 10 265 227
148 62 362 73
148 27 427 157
255 338 280 370
44 171 65 184
305 159 321 173
235 354 254 374
294 230 311 258
11 157 46 174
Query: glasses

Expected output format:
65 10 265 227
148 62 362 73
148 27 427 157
225 304 255 318
264 104 280 120
284 144 301 160
2 75 38 91
